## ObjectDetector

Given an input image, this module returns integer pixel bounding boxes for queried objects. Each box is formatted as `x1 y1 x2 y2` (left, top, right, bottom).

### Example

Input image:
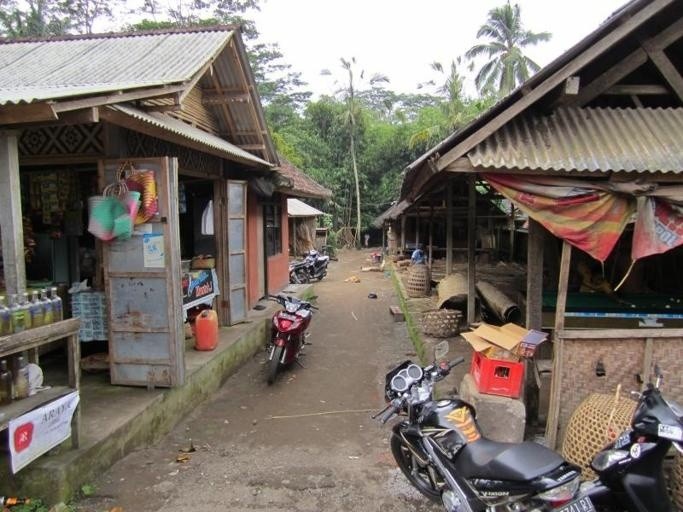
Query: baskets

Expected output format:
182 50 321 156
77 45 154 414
87 184 143 243
669 448 683 509
191 255 215 269
406 265 432 298
420 309 463 339
562 392 638 480
116 161 158 226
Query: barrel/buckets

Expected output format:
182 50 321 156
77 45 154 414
195 310 218 350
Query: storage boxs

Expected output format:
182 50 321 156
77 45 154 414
471 350 524 399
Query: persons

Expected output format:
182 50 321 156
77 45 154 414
569 261 623 306
363 231 370 248
408 242 429 267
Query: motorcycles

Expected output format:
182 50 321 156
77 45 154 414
290 262 310 285
264 294 319 385
370 358 584 512
553 364 678 510
302 249 329 280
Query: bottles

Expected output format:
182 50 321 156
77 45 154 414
0 360 12 406
0 287 63 335
0 495 31 508
13 357 29 399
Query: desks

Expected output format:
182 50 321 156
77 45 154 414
0 316 82 449
